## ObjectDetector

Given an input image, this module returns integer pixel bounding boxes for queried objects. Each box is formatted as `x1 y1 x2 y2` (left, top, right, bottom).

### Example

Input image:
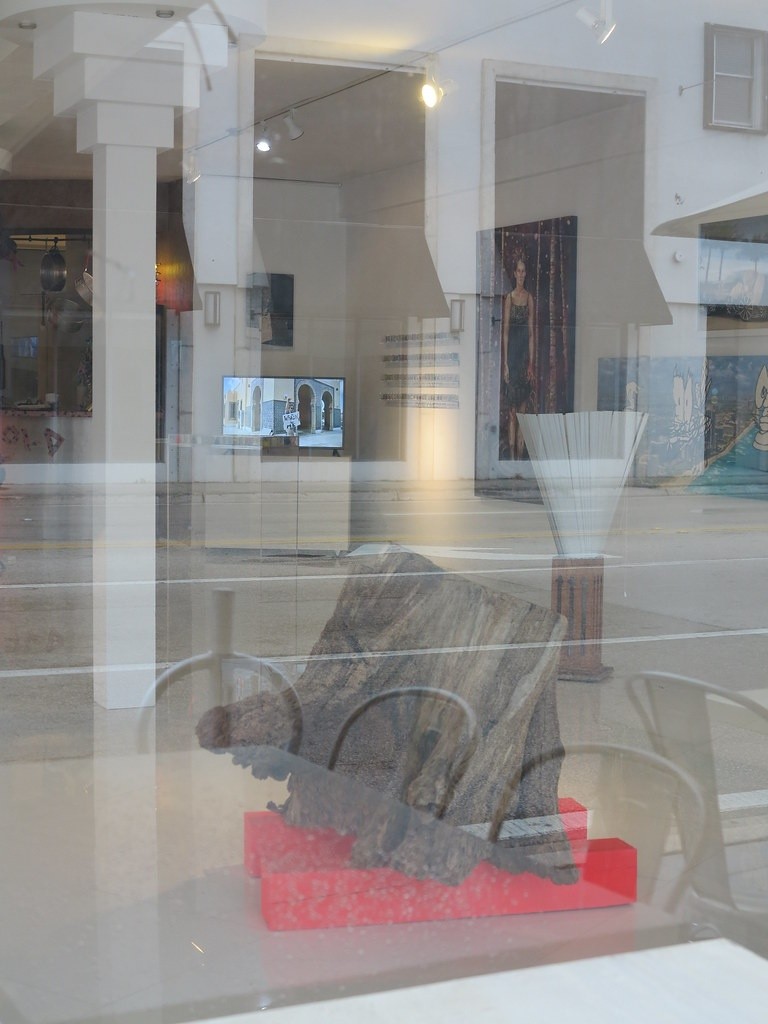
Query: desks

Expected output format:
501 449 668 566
0 741 691 1024
189 937 768 1023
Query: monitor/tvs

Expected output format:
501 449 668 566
221 376 347 449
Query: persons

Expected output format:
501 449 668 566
504 250 535 478
285 398 296 435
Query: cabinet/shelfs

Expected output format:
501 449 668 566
202 454 350 557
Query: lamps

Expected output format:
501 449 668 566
576 0 618 49
256 120 273 152
421 55 456 108
283 108 305 142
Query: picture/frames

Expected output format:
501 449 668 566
473 216 576 506
694 205 767 339
244 272 295 351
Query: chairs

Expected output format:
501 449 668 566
133 654 304 752
328 687 479 817
488 743 710 918
624 672 768 962
